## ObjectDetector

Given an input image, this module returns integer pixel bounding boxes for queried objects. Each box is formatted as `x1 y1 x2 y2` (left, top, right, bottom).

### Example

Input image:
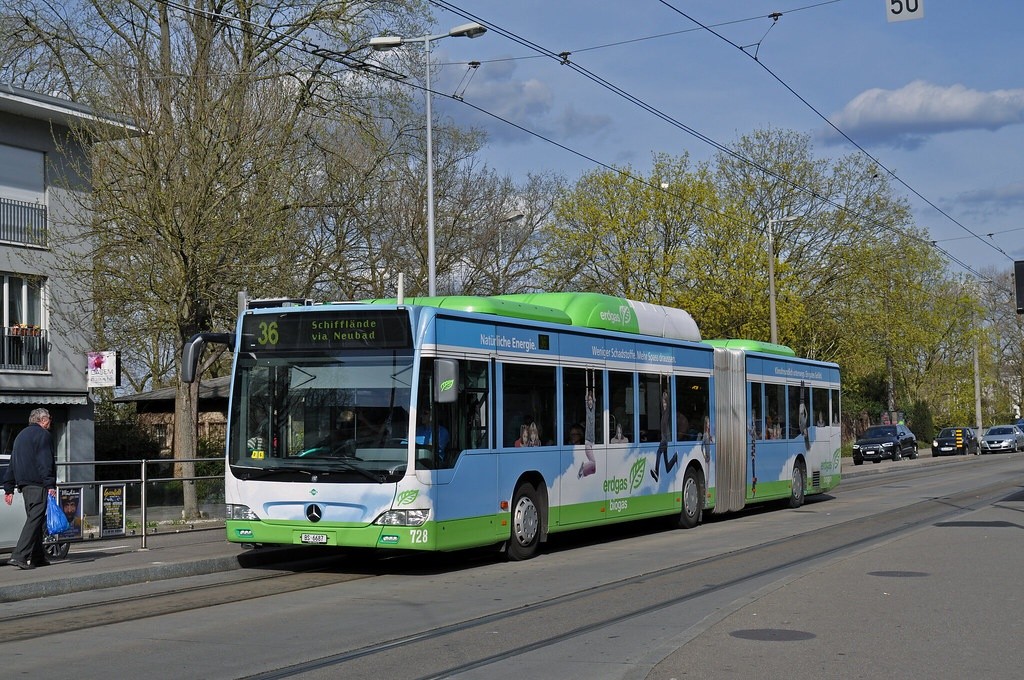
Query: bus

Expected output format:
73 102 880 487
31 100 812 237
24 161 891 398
180 290 843 561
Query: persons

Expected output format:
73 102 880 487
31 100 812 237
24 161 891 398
61 494 80 536
677 408 689 440
399 404 450 468
771 424 782 439
305 411 359 455
612 424 628 443
578 365 596 478
515 422 541 447
650 372 679 482
799 380 811 450
701 416 713 506
833 413 839 426
569 424 585 445
4 408 57 570
817 411 825 427
747 410 756 498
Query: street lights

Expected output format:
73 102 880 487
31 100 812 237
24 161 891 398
767 214 798 345
370 21 489 295
495 211 526 296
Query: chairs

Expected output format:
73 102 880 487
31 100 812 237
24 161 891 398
947 431 952 436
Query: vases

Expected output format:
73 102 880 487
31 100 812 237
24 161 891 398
27 329 33 335
12 327 19 335
21 329 26 336
36 330 41 335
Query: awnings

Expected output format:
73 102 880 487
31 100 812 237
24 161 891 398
0 395 87 405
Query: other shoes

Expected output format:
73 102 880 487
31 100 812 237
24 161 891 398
7 557 32 570
30 560 50 567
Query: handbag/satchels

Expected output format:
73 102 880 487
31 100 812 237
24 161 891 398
46 491 70 535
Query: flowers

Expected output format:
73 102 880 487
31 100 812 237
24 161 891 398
13 321 40 328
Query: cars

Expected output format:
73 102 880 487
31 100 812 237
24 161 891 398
980 425 1024 452
1011 419 1024 431
932 427 981 456
0 453 73 563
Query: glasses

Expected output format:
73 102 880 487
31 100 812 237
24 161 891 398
43 415 53 422
423 410 429 415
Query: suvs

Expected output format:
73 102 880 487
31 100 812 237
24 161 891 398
852 424 919 466
